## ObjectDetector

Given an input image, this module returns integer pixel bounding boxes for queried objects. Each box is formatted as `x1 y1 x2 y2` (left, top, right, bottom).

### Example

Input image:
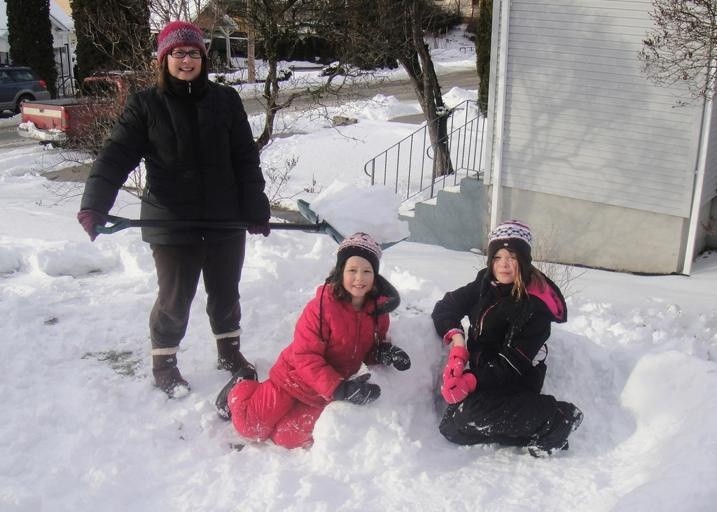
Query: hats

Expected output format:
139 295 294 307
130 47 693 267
167 50 203 59
336 232 382 276
488 219 532 247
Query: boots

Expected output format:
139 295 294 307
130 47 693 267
214 329 255 376
215 367 258 421
528 401 584 458
153 346 190 398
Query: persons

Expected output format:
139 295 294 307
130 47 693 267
215 232 410 447
78 21 271 399
431 217 584 458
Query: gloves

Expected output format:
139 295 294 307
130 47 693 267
248 219 270 237
343 373 380 405
77 210 107 241
441 346 477 405
376 342 411 371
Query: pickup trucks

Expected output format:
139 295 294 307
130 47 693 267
17 73 126 155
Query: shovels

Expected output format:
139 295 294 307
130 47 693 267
94 199 412 249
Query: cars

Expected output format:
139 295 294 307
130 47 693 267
0 67 51 114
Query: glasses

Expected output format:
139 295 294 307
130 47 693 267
157 22 207 62
